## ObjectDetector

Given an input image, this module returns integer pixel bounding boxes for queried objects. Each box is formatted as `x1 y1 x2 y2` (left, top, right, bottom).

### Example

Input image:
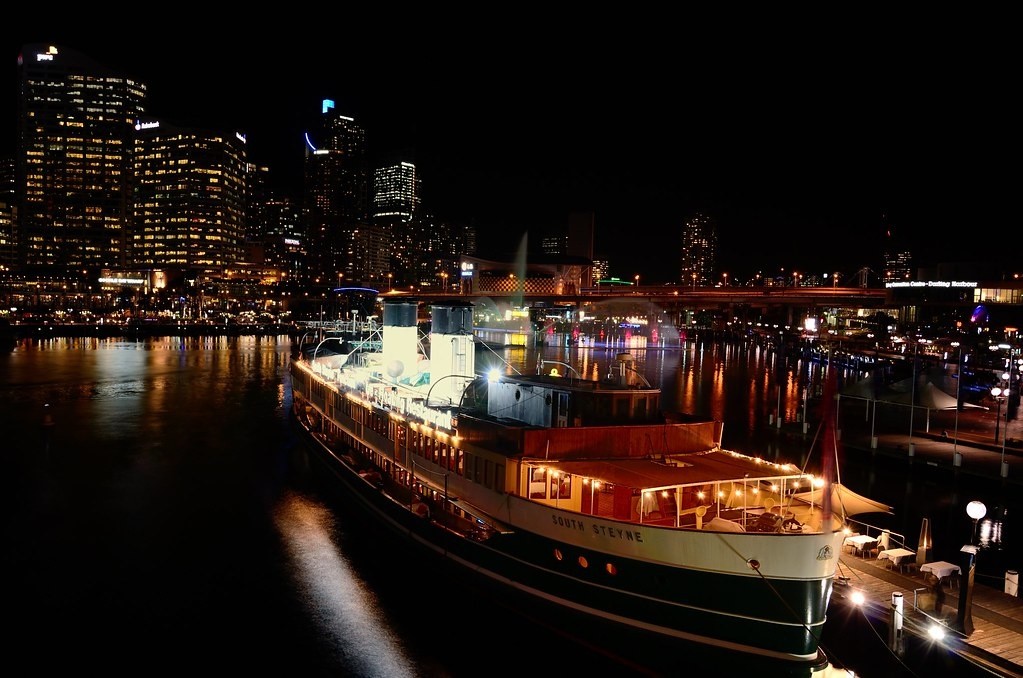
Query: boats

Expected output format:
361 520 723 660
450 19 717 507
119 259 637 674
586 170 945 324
287 294 852 672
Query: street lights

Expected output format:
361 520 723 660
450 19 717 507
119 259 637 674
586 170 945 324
956 501 986 638
991 386 1010 443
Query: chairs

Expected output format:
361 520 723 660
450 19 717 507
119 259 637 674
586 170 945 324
749 513 782 532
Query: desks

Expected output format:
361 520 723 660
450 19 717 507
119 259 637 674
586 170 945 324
920 560 962 590
843 534 878 558
878 548 916 574
